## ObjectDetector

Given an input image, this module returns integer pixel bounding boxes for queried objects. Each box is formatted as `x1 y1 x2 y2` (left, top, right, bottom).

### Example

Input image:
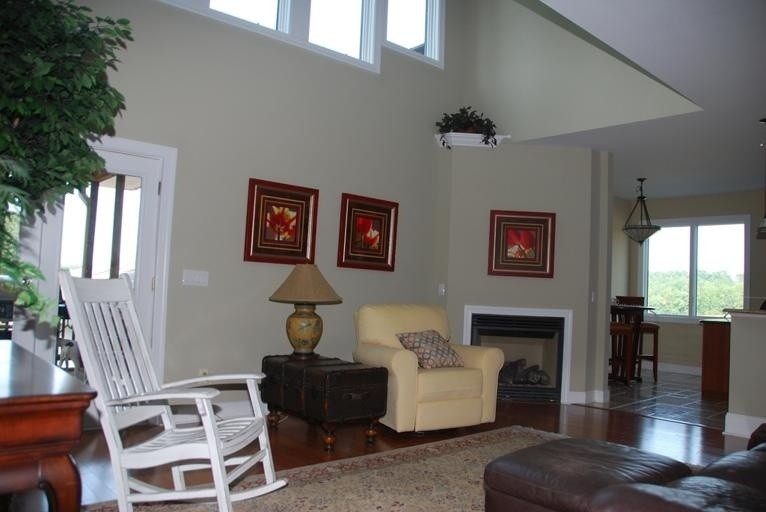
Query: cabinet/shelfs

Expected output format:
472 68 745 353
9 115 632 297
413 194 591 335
701 319 730 403
723 308 765 451
263 355 388 452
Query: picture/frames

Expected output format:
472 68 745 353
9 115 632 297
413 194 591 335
488 209 555 277
337 192 399 272
243 178 319 263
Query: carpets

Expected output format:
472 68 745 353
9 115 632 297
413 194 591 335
67 426 571 512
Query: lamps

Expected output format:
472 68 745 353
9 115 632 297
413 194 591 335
622 179 661 245
270 264 343 359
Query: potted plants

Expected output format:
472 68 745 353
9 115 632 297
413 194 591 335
436 105 498 149
0 2 134 338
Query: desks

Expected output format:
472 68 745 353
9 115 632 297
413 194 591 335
1 339 98 511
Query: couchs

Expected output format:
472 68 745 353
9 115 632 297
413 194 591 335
581 422 765 511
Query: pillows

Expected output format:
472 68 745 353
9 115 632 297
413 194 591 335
397 331 463 369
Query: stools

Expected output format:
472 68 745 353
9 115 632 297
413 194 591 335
484 436 692 512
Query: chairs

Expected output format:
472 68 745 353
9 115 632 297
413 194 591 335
610 295 658 383
58 268 289 512
353 304 505 433
